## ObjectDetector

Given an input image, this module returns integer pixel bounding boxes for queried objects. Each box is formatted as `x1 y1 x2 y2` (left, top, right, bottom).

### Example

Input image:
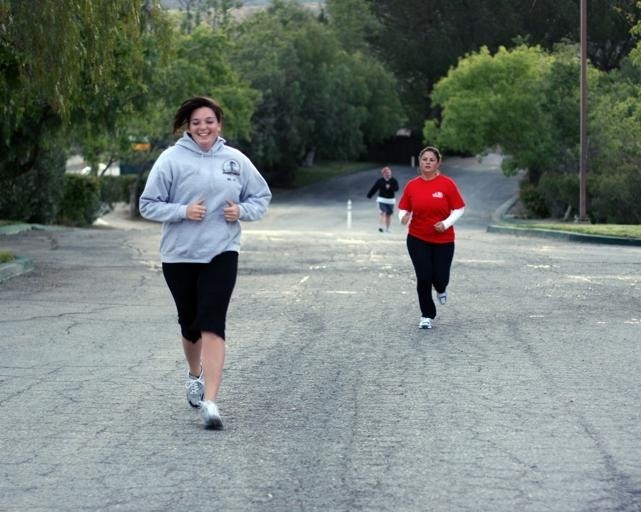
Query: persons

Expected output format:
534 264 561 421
397 146 466 329
138 96 272 429
366 166 399 233
225 161 239 173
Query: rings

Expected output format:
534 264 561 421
199 215 202 218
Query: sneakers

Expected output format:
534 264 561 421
196 399 223 429
186 365 204 408
437 289 448 305
379 227 394 234
418 316 432 329
